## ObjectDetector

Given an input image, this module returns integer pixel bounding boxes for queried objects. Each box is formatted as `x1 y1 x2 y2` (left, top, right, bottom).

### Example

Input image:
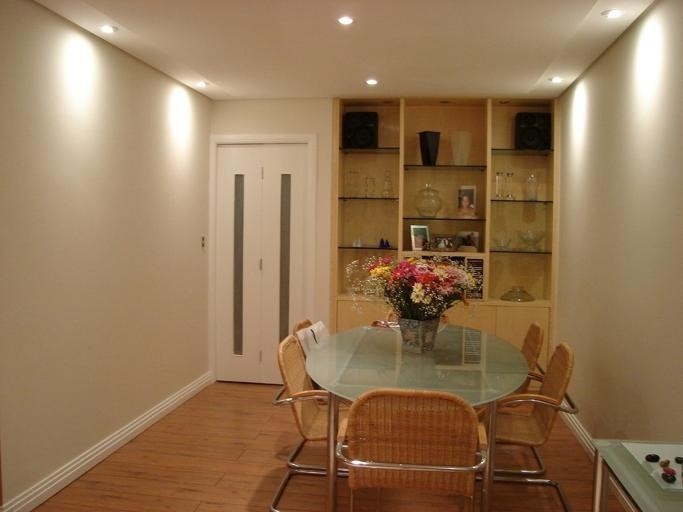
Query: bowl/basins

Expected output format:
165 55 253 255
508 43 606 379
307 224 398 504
491 238 512 249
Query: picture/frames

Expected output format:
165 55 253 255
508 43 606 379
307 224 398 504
410 225 430 251
456 186 477 219
331 96 561 391
431 234 456 252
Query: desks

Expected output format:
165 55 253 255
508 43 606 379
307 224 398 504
592 439 683 512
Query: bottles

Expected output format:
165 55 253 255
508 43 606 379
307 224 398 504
526 174 536 202
414 182 441 218
363 177 376 197
504 170 514 199
494 170 502 198
382 170 394 196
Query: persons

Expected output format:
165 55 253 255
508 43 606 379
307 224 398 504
460 195 476 211
415 229 429 246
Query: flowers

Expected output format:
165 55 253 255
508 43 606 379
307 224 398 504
345 256 483 326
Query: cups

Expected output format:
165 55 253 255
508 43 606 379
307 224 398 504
450 129 472 166
419 130 439 166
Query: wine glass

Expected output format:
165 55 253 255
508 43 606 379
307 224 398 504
516 228 545 252
